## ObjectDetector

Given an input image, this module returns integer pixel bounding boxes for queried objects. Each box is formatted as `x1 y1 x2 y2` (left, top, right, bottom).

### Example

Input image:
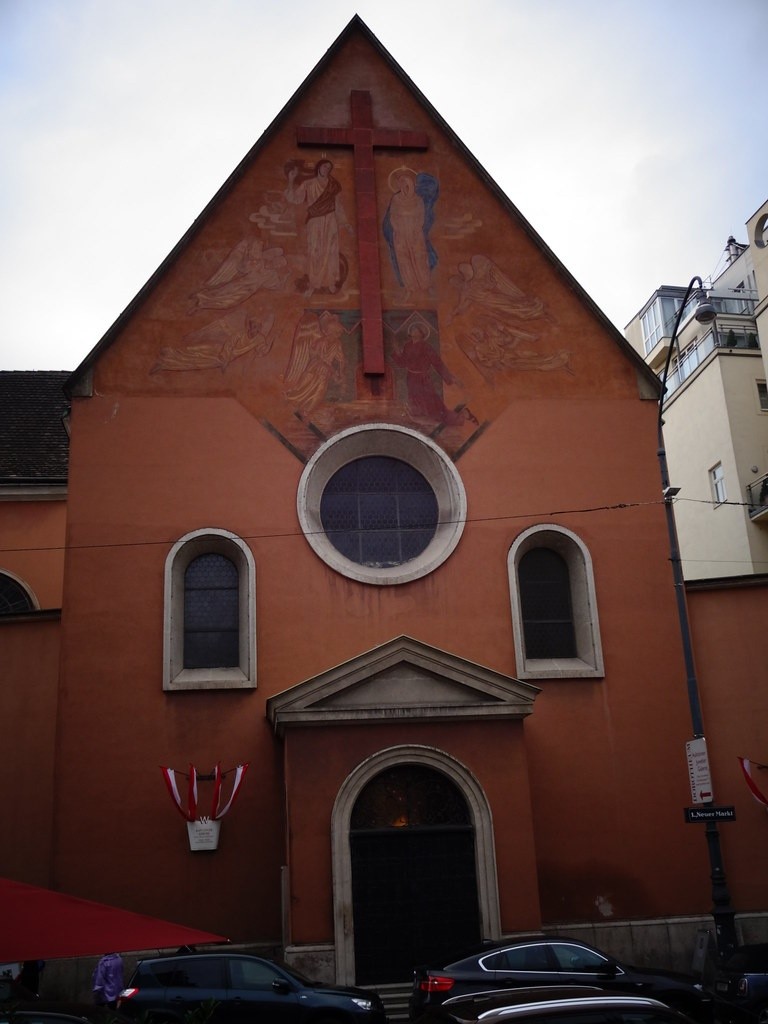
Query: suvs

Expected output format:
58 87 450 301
113 949 389 1024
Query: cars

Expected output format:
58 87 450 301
0 1000 137 1024
406 933 768 1024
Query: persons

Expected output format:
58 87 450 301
15 960 46 993
91 952 125 1010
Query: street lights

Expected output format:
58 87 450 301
654 274 744 967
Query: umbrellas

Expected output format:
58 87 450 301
0 876 232 965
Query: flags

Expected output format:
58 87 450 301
736 755 768 806
159 759 251 822
